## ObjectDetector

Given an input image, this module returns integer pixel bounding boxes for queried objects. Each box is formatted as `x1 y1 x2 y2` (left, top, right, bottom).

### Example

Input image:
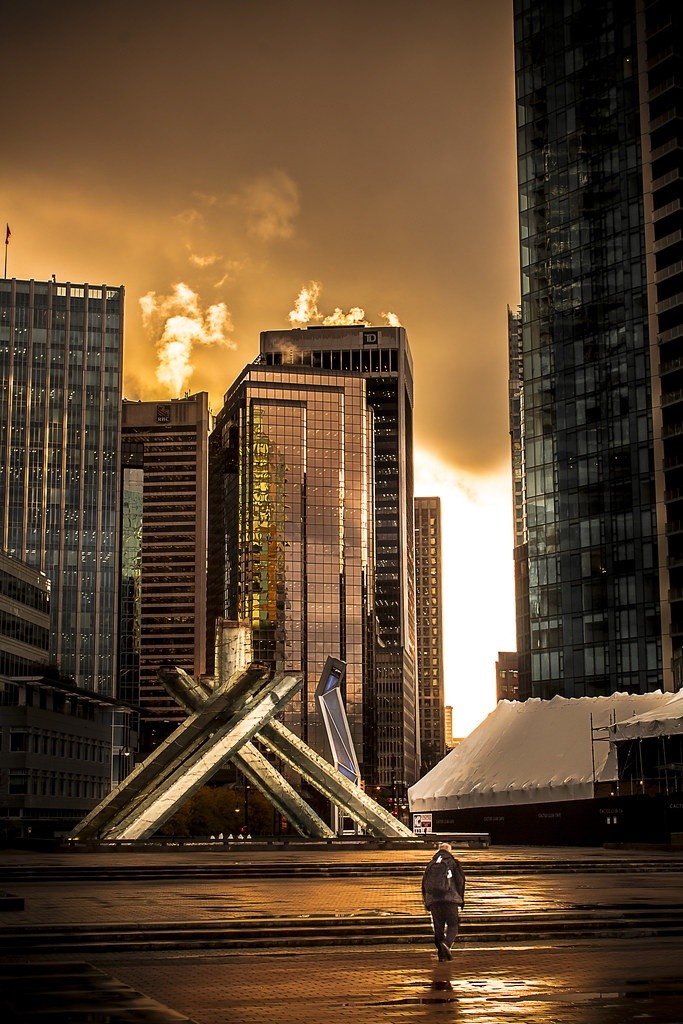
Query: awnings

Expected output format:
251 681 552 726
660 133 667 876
408 687 683 813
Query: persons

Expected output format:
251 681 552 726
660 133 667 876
422 843 466 961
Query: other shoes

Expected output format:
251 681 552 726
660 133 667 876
439 956 445 961
440 940 452 961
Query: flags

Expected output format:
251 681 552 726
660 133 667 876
5 225 10 244
